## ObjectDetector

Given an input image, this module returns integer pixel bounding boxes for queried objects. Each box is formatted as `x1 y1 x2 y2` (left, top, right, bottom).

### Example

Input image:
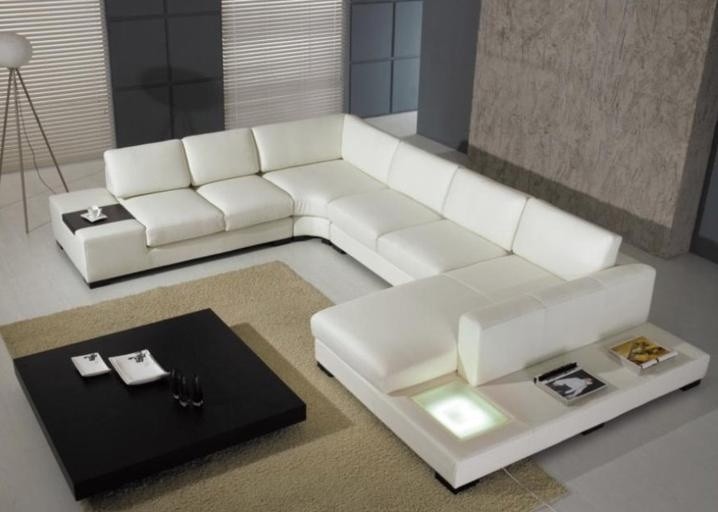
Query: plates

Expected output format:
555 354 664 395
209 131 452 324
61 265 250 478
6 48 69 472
108 349 169 386
80 212 108 222
71 352 112 377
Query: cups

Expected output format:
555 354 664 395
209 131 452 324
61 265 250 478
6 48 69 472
87 206 103 218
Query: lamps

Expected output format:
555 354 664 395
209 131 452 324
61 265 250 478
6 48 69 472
0 32 70 235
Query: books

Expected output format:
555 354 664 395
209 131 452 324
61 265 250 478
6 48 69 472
605 334 680 376
533 361 609 405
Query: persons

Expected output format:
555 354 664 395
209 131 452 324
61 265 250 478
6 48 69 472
550 376 594 400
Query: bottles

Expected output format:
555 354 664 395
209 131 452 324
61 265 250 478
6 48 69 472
170 368 204 407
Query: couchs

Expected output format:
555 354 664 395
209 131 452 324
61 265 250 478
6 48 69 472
48 111 709 491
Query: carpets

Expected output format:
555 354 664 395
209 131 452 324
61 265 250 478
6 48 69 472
0 261 571 512
